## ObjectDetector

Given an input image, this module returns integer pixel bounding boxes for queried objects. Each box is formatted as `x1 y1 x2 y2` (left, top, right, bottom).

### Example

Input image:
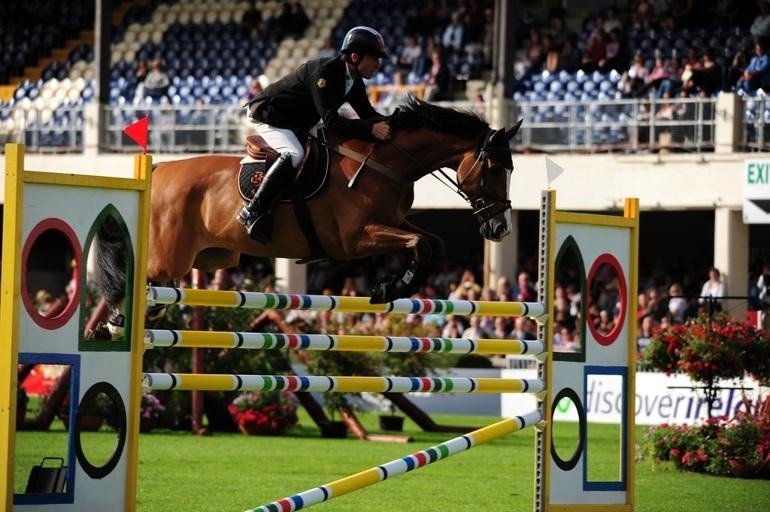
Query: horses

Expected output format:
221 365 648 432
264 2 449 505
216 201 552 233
88 92 524 343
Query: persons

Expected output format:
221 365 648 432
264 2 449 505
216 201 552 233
236 26 391 244
134 59 175 151
241 1 311 40
192 100 209 125
224 263 769 352
400 0 770 153
320 38 337 59
239 82 263 107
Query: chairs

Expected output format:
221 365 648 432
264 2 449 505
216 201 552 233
0 1 769 147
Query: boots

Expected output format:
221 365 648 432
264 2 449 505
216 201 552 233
237 154 298 245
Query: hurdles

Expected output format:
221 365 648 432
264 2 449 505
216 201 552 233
0 141 639 511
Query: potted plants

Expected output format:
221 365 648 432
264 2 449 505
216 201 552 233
303 330 383 439
365 317 453 432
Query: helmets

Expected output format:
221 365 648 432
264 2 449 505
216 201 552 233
340 26 390 60
